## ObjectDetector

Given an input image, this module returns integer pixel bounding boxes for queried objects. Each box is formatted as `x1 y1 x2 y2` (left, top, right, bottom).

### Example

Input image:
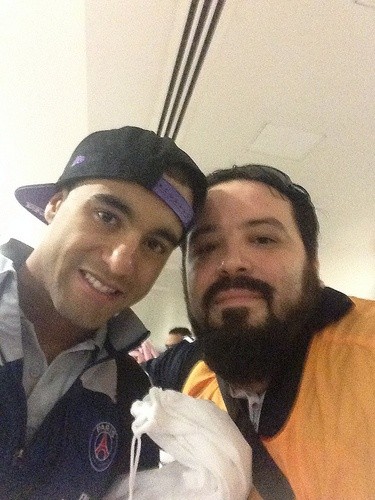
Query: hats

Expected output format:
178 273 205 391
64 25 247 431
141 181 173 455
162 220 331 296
14 125 207 236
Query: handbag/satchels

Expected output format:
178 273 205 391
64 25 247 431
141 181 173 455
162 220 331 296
106 386 257 500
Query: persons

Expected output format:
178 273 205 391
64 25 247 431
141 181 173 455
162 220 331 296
0 123 207 499
134 164 375 500
164 327 192 353
